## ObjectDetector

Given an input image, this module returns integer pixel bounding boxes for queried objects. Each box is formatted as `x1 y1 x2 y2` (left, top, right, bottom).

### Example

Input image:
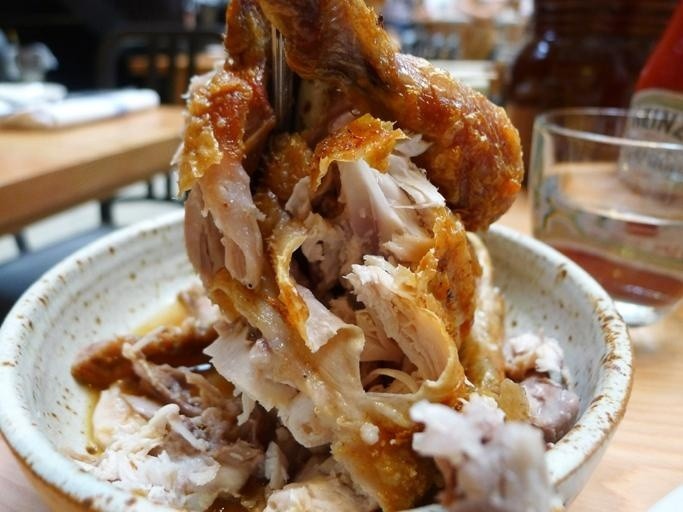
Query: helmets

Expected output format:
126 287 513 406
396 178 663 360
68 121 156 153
1 197 637 512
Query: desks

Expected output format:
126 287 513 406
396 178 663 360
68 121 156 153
1 162 683 511
2 105 185 254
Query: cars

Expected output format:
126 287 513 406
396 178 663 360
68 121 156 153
522 90 683 329
